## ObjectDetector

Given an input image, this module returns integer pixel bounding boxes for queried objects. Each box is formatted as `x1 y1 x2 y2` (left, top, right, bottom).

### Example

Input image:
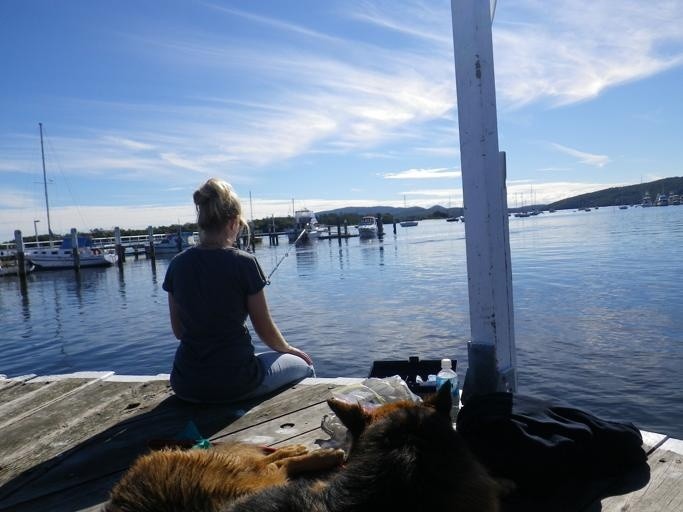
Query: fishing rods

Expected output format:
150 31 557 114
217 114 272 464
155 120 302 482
265 230 305 285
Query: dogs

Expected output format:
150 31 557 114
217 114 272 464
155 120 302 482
98 379 517 512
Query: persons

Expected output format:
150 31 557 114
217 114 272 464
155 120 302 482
161 178 315 406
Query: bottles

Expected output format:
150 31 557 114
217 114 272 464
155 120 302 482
436 358 461 405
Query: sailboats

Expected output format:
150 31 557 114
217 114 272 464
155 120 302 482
504 184 541 217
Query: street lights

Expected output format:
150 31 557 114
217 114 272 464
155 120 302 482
32 219 40 247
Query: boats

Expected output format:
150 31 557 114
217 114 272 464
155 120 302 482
287 208 320 241
360 236 383 244
548 206 599 213
289 239 317 255
30 267 107 295
0 244 24 259
145 251 183 260
617 191 682 211
145 231 197 252
399 221 419 227
356 216 379 237
22 232 117 270
444 216 464 223
